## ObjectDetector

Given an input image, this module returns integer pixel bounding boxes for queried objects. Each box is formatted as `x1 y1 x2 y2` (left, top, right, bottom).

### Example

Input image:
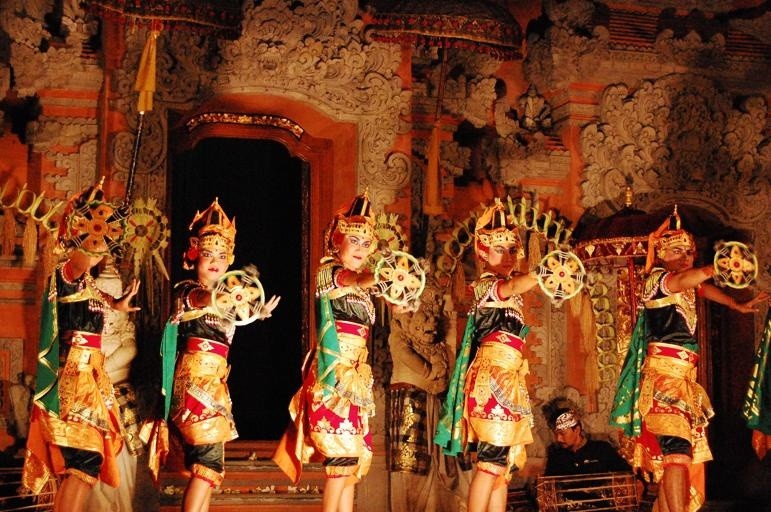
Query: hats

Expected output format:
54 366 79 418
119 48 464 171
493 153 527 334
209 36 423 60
50 174 111 255
327 184 380 255
182 196 238 270
546 406 580 434
472 196 528 264
646 203 700 260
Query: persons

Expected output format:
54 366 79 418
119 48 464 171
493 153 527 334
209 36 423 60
94 279 147 512
138 194 283 512
608 203 771 511
22 176 143 512
435 198 600 512
538 408 650 511
271 186 424 512
